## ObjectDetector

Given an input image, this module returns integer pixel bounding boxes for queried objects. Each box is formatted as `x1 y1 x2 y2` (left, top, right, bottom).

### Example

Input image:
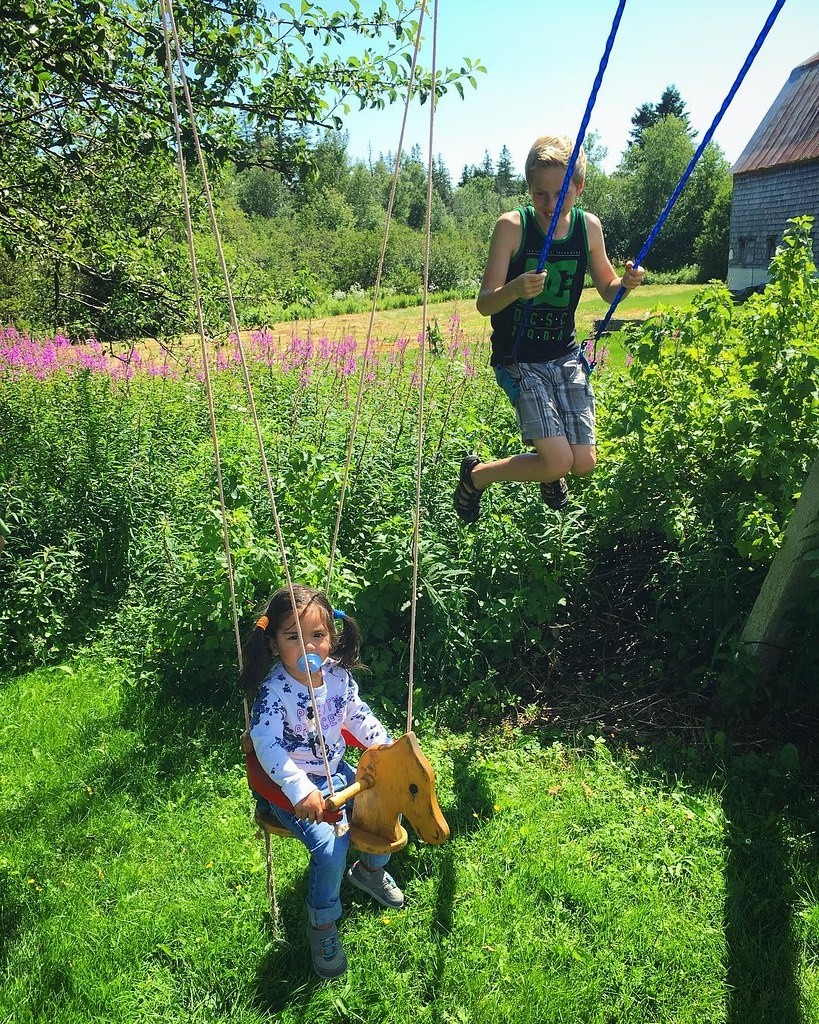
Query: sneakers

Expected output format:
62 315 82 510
347 860 405 908
454 455 483 521
531 448 568 509
307 918 347 979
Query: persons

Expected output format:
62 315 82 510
238 584 405 979
453 135 645 524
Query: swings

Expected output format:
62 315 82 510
494 0 786 407
164 0 454 858
